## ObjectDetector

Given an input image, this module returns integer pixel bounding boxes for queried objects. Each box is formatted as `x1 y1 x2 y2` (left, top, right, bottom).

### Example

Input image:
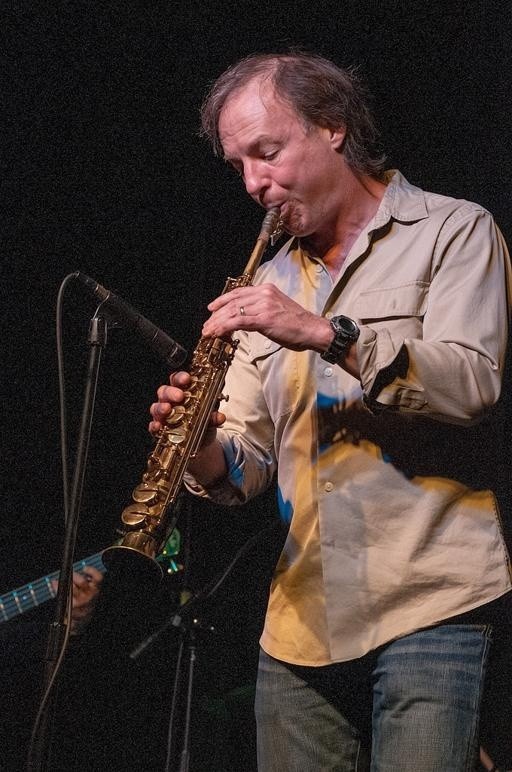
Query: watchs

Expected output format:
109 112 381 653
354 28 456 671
320 315 360 365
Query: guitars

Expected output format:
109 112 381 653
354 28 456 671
0 527 180 623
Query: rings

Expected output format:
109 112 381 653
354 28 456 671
240 305 246 316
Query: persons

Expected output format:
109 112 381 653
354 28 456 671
148 45 512 772
0 566 105 667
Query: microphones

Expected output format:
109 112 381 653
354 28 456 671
73 270 188 370
